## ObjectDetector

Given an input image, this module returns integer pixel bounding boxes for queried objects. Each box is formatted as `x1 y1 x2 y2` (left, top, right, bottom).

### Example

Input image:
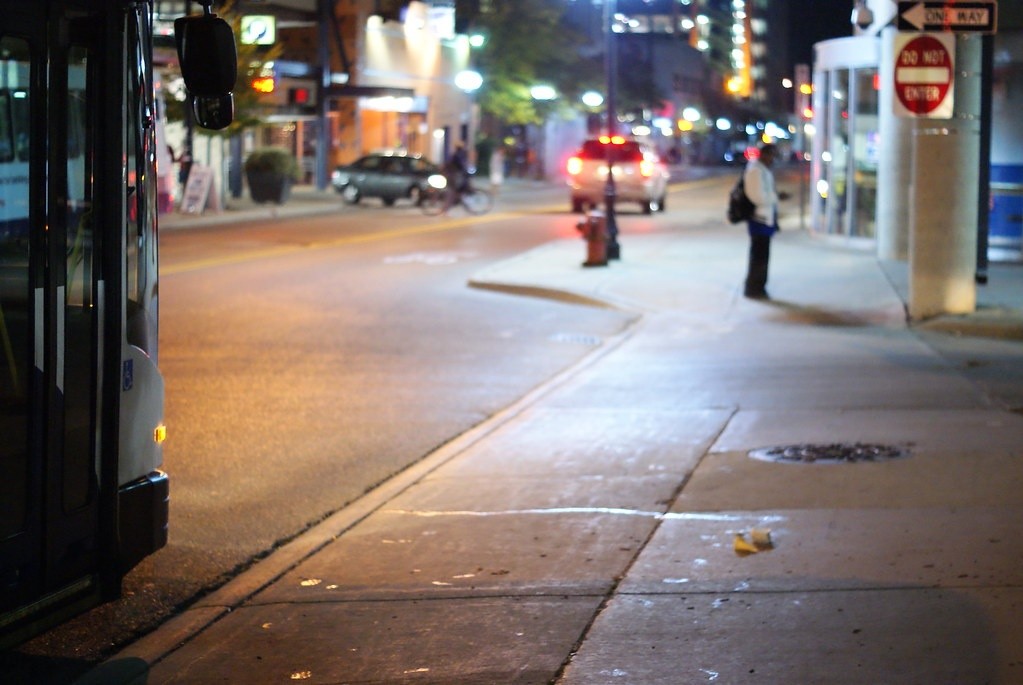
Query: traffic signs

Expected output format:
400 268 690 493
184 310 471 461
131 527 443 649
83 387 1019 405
896 0 997 37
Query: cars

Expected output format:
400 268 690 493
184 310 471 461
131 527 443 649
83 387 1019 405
568 137 673 215
335 149 452 207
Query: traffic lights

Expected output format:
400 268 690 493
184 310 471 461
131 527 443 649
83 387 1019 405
288 86 313 107
251 77 277 94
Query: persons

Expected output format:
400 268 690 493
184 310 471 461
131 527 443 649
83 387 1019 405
741 143 783 297
431 140 475 209
168 143 193 192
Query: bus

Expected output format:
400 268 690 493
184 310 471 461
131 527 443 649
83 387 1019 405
0 84 92 250
0 0 239 654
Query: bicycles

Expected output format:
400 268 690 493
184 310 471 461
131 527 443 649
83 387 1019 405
417 168 495 217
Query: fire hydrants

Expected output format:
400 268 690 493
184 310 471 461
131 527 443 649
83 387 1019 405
577 211 612 268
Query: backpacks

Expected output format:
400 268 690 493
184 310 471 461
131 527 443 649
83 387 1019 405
728 160 757 223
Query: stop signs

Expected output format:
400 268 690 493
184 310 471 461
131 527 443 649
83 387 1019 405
891 33 957 121
